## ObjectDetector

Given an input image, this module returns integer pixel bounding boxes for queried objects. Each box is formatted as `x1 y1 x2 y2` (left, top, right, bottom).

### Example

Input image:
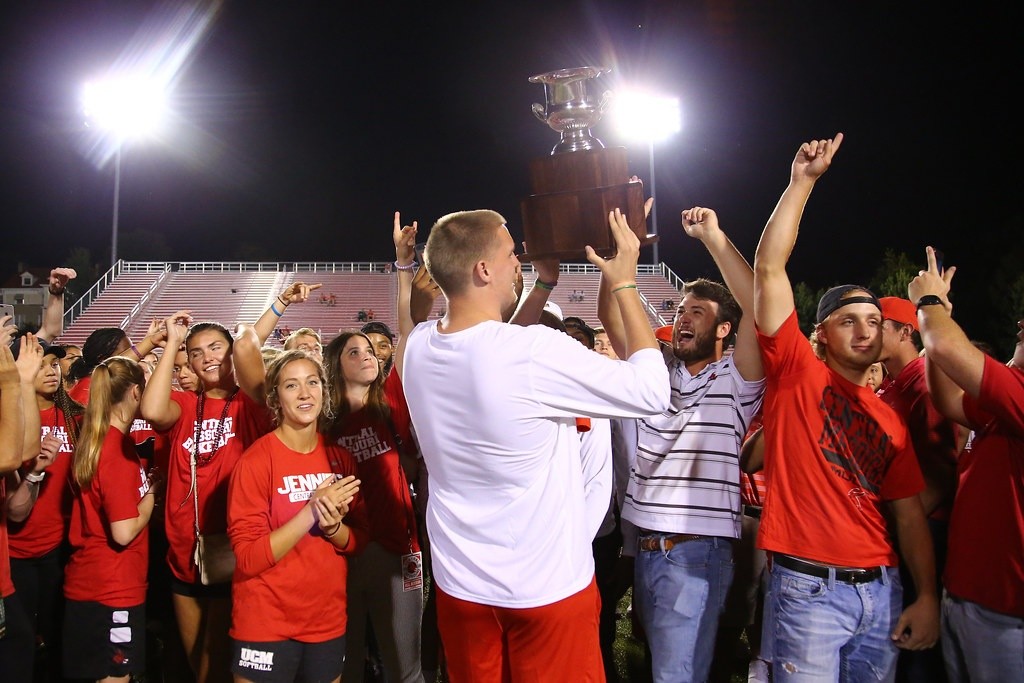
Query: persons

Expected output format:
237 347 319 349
0 131 1023 683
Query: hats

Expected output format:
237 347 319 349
361 322 396 344
9 336 67 363
816 284 882 324
876 297 920 332
542 300 565 326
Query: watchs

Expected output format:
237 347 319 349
915 295 945 315
25 471 45 482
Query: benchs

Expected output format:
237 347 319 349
51 272 681 346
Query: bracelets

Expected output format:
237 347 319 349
146 493 155 494
131 345 145 360
534 279 554 291
322 519 341 539
612 284 637 292
271 304 282 317
278 296 288 307
395 260 416 270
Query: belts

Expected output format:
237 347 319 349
637 533 706 551
742 504 762 520
772 550 892 585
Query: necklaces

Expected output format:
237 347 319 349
194 384 239 466
